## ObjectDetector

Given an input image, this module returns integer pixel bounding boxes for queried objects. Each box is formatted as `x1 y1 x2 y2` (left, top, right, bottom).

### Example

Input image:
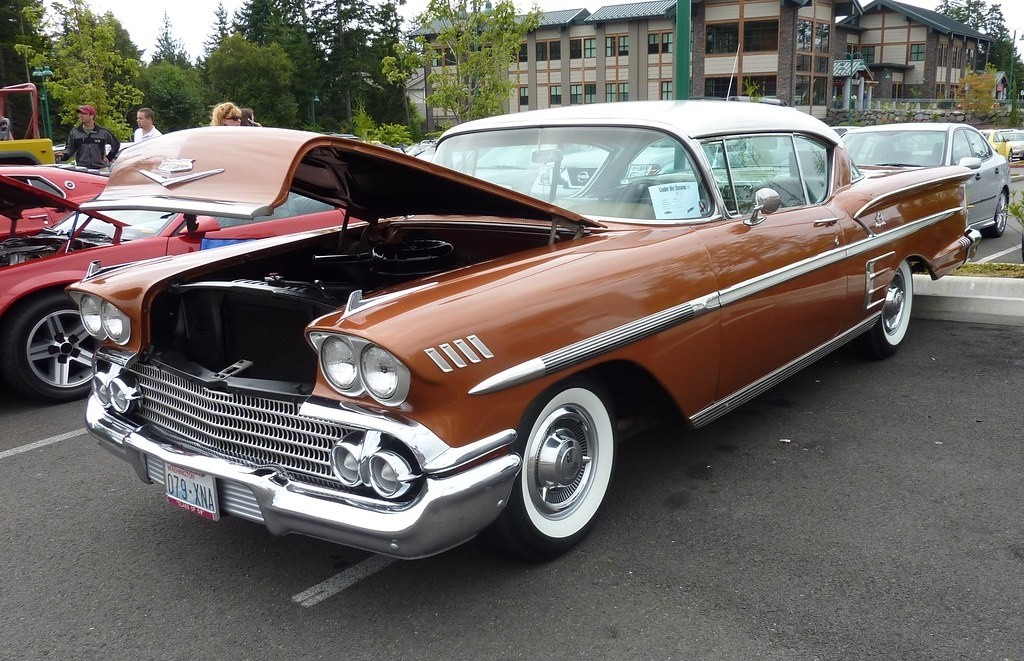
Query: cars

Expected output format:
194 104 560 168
64 101 983 565
53 144 67 151
832 123 1024 238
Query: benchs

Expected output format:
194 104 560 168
894 150 971 166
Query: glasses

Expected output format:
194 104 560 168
224 116 241 120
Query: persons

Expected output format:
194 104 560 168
133 106 162 143
54 104 120 171
1 115 14 139
209 100 263 127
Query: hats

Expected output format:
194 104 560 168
76 105 97 116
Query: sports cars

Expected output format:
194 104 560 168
0 164 367 402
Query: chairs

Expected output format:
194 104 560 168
619 179 670 204
645 173 719 205
751 174 825 212
868 141 899 164
926 142 960 166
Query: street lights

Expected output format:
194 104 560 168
839 45 863 121
1005 29 1024 98
32 66 54 140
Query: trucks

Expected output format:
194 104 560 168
0 82 55 164
